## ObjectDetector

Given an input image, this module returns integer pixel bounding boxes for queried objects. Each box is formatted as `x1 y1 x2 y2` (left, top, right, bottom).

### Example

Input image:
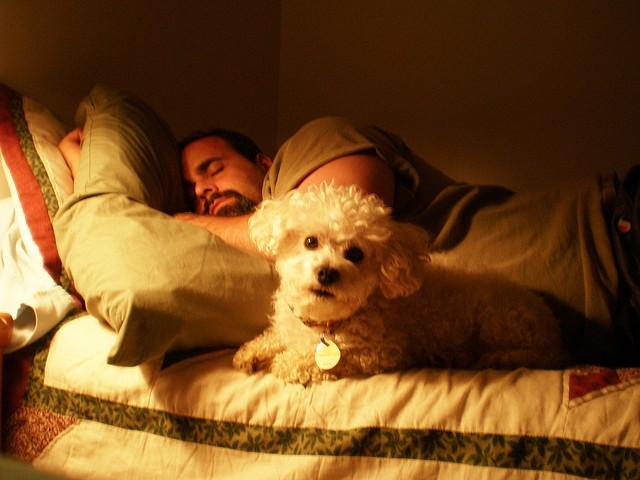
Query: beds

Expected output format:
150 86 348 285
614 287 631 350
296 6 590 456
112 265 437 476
0 80 640 479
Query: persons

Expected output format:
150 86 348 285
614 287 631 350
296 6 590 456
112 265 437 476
54 114 639 369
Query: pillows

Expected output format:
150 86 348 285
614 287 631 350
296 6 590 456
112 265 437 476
53 83 279 370
0 85 85 293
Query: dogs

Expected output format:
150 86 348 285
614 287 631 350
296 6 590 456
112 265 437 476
233 178 570 387
